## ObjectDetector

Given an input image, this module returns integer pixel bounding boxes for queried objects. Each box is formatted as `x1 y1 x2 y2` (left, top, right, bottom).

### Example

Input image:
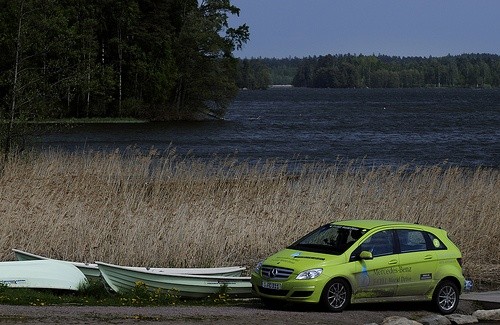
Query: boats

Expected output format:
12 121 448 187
11 248 246 289
0 260 91 291
94 260 256 301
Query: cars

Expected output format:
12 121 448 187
248 219 465 315
